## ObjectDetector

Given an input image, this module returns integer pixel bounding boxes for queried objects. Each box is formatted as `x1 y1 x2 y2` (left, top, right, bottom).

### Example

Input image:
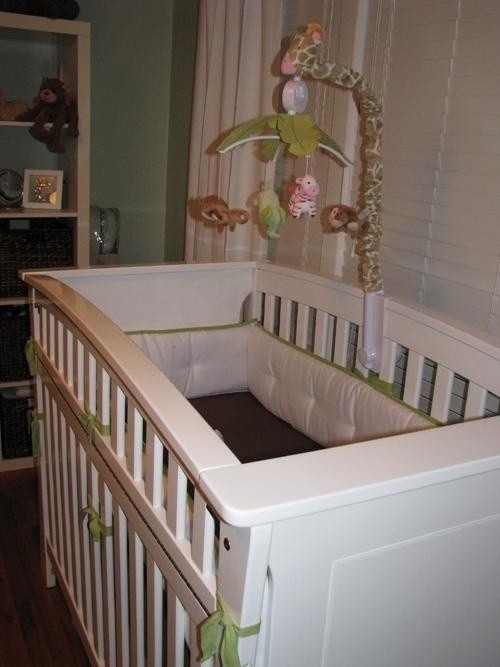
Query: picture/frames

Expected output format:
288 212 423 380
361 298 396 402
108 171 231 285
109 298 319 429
21 168 65 210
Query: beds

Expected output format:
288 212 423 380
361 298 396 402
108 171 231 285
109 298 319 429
17 261 500 665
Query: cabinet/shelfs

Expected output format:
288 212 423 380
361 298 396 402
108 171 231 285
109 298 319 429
1 12 92 474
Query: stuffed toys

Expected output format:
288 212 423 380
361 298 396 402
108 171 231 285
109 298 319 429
187 21 382 292
186 22 384 291
17 76 79 139
0 89 25 121
